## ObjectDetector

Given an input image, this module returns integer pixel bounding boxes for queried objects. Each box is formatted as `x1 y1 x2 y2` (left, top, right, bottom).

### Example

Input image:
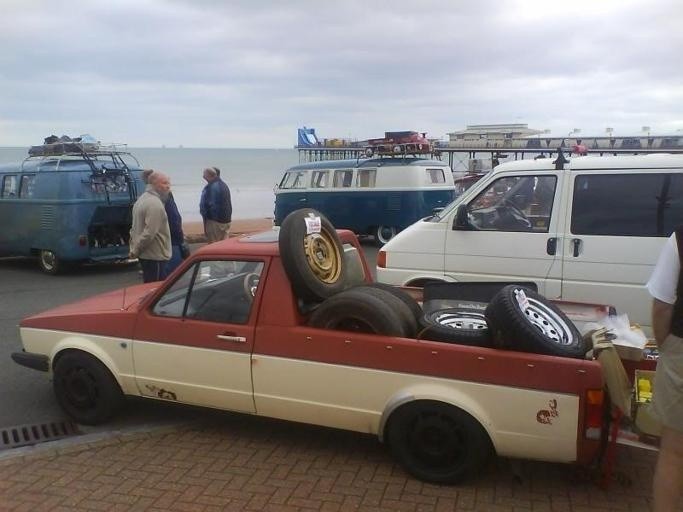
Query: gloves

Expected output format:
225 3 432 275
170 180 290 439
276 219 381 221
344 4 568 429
180 241 190 260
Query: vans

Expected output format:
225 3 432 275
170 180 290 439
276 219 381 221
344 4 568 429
374 148 683 351
0 135 151 274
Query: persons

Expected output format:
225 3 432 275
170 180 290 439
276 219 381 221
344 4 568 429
642 224 683 512
572 138 587 154
200 166 220 241
127 171 173 284
203 166 232 244
142 166 185 275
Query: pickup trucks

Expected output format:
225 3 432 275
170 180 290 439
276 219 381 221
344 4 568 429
11 230 660 483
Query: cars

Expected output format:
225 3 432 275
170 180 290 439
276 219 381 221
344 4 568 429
454 172 518 208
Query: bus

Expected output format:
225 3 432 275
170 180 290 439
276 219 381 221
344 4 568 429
272 146 455 248
